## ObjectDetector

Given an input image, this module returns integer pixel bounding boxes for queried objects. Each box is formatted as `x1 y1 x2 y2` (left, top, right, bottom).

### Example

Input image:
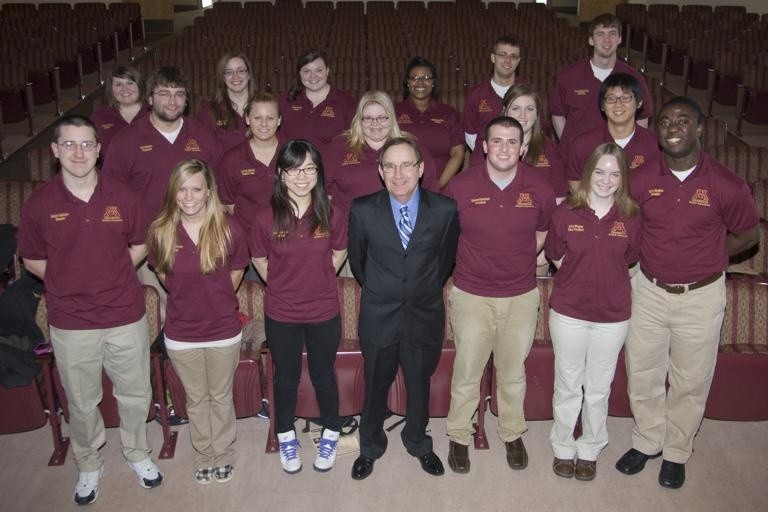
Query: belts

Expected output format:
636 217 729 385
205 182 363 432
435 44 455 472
639 263 724 295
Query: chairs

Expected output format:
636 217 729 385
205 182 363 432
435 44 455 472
164 280 279 453
726 222 768 275
704 279 768 421
1 3 146 137
608 278 633 417
491 276 554 421
386 277 489 448
34 285 177 459
0 293 70 466
294 277 366 418
617 4 768 138
708 146 768 183
31 145 57 180
752 180 768 222
0 181 46 292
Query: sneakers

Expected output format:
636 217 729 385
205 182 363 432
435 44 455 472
192 465 216 485
72 463 105 507
276 429 303 475
127 456 164 489
313 428 341 473
213 465 234 484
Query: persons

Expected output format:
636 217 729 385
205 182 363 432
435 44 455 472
392 57 466 189
439 119 558 471
102 65 225 215
318 91 438 212
143 160 251 482
614 94 759 488
192 52 255 152
276 48 357 142
216 92 293 219
89 64 153 169
470 79 566 195
248 139 345 473
548 13 654 142
15 115 162 505
463 37 523 150
543 143 646 481
566 72 657 197
345 136 459 481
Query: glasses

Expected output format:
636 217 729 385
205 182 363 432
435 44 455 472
602 93 636 104
382 160 420 174
222 69 249 76
409 74 433 82
281 167 319 176
495 52 521 61
361 116 389 123
154 90 187 99
56 140 98 152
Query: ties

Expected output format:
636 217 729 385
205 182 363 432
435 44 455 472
398 204 413 253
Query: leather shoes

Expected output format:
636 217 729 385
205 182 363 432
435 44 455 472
351 448 386 480
574 457 597 481
407 447 445 476
615 447 662 475
448 439 471 474
505 436 528 471
658 458 686 490
552 456 575 479
